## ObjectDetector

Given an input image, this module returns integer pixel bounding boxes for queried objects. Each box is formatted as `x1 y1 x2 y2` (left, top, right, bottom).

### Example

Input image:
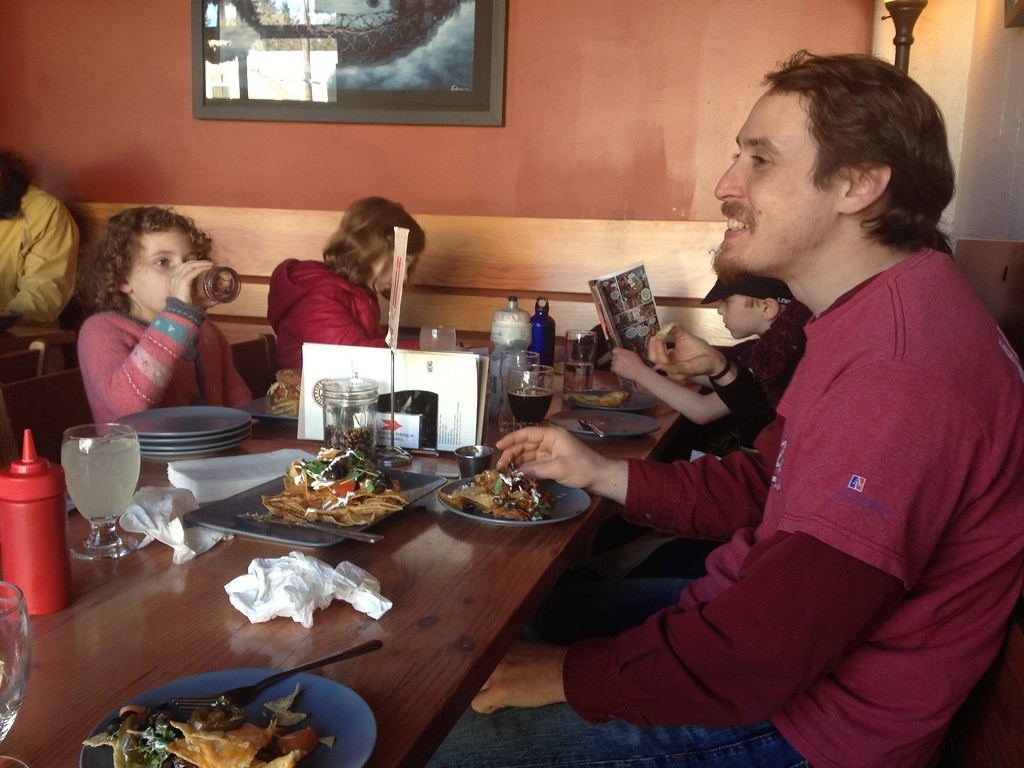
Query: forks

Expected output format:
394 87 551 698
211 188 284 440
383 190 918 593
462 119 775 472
170 639 383 711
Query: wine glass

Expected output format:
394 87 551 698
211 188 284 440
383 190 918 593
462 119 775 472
0 580 32 768
63 422 138 558
508 364 554 432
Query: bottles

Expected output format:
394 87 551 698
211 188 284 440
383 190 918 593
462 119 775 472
528 296 555 369
322 379 378 459
0 427 77 618
488 295 532 420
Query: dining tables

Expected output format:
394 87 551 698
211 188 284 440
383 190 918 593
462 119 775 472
0 343 704 768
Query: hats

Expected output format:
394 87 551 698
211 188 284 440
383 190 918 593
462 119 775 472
700 270 794 305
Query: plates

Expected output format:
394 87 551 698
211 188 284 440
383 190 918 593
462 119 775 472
438 473 592 527
566 389 663 411
238 393 301 422
111 406 254 465
551 410 662 439
183 456 448 549
80 666 377 767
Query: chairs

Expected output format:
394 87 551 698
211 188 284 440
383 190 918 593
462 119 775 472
0 331 274 467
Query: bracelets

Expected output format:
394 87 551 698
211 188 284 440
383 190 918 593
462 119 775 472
707 357 731 380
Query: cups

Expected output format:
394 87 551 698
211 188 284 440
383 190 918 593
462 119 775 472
561 330 594 404
498 351 540 439
418 325 456 353
190 265 241 308
454 445 495 481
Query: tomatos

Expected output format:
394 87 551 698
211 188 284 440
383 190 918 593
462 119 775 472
275 727 319 760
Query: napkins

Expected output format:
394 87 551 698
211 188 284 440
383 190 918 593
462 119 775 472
167 449 319 505
222 552 394 629
119 485 232 565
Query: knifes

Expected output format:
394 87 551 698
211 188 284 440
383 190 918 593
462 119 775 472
232 513 384 543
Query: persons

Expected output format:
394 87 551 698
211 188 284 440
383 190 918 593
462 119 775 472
267 195 427 368
77 207 252 436
413 49 1024 768
0 149 81 330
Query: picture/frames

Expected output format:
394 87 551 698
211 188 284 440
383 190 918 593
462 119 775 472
192 1 507 126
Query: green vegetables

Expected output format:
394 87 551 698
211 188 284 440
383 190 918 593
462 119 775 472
105 703 183 768
493 462 553 516
300 449 380 494
